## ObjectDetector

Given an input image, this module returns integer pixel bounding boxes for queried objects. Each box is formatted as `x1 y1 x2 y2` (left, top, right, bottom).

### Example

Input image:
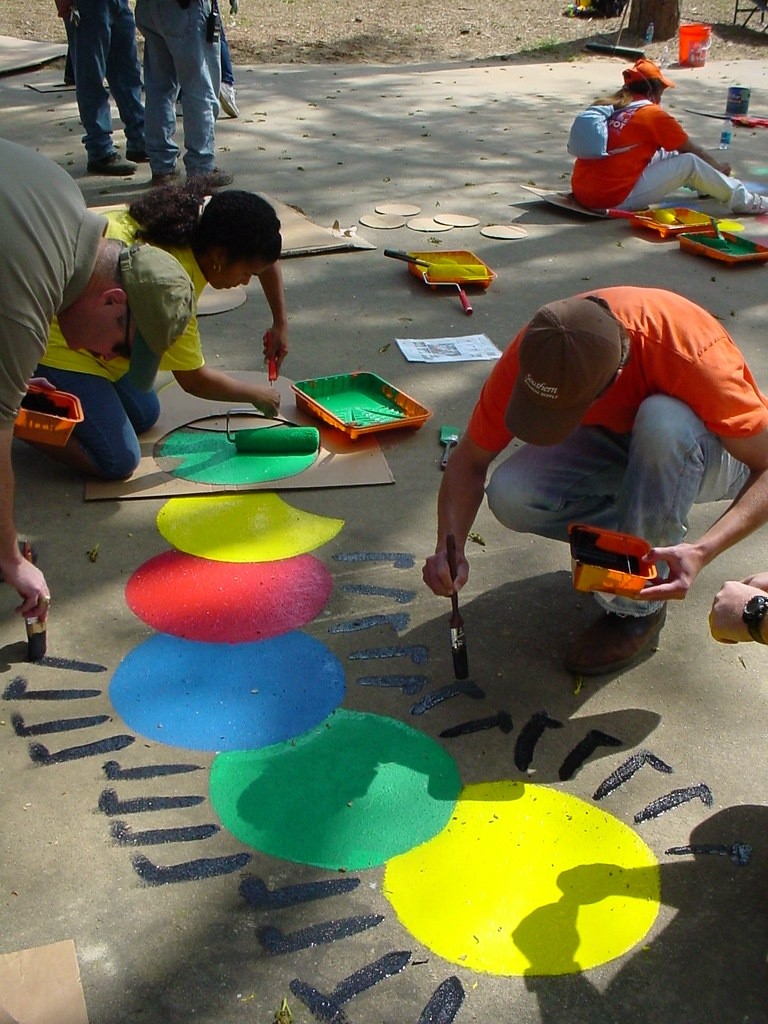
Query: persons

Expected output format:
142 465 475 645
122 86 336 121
54 0 239 184
709 571 768 644
571 60 768 213
0 136 197 626
19 168 287 481
422 285 768 674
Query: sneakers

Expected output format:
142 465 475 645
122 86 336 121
218 82 239 117
734 193 768 214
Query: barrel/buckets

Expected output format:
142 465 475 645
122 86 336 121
679 24 712 68
725 77 752 115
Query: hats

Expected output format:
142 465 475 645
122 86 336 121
623 60 677 89
505 298 622 447
120 243 194 395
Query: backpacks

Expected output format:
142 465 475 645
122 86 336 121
566 100 656 160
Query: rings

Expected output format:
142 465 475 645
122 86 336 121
43 595 51 609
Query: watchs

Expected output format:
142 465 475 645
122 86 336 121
742 595 768 645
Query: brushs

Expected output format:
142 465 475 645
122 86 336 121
585 0 645 58
710 216 726 242
445 532 471 681
439 424 460 470
226 407 319 455
604 208 676 226
422 264 487 315
23 539 47 662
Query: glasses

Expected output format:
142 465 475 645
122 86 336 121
112 307 131 360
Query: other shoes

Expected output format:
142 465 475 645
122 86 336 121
566 598 667 674
126 148 181 162
188 168 234 187
87 153 136 175
151 163 181 186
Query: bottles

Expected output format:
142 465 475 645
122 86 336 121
660 45 669 70
719 117 733 150
644 23 654 45
